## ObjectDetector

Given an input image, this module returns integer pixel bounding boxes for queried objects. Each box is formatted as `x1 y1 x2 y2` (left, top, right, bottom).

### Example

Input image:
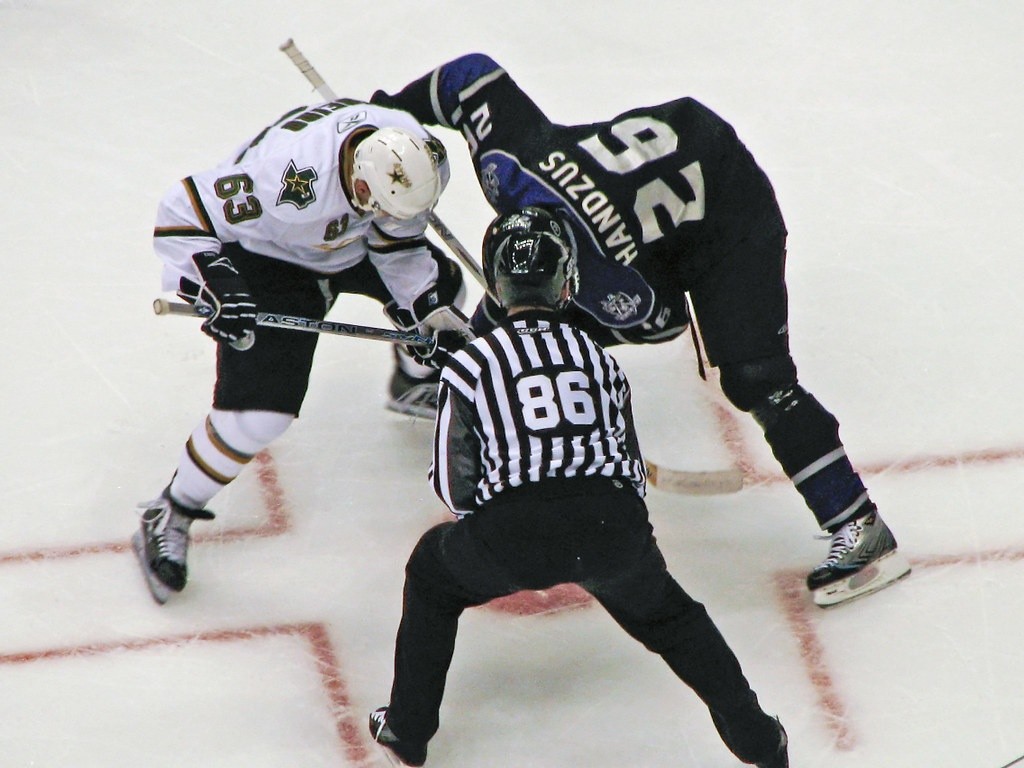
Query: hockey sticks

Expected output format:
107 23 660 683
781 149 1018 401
278 37 503 307
643 460 745 498
153 297 432 348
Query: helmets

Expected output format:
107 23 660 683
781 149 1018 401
482 206 578 307
351 126 441 220
493 232 575 312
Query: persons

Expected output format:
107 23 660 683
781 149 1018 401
369 53 913 610
369 205 789 768
131 98 477 607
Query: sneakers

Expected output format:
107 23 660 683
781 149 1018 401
806 510 911 608
369 706 427 768
385 345 439 422
754 717 789 768
131 468 215 605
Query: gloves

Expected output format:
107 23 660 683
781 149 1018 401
384 283 477 370
178 250 255 343
